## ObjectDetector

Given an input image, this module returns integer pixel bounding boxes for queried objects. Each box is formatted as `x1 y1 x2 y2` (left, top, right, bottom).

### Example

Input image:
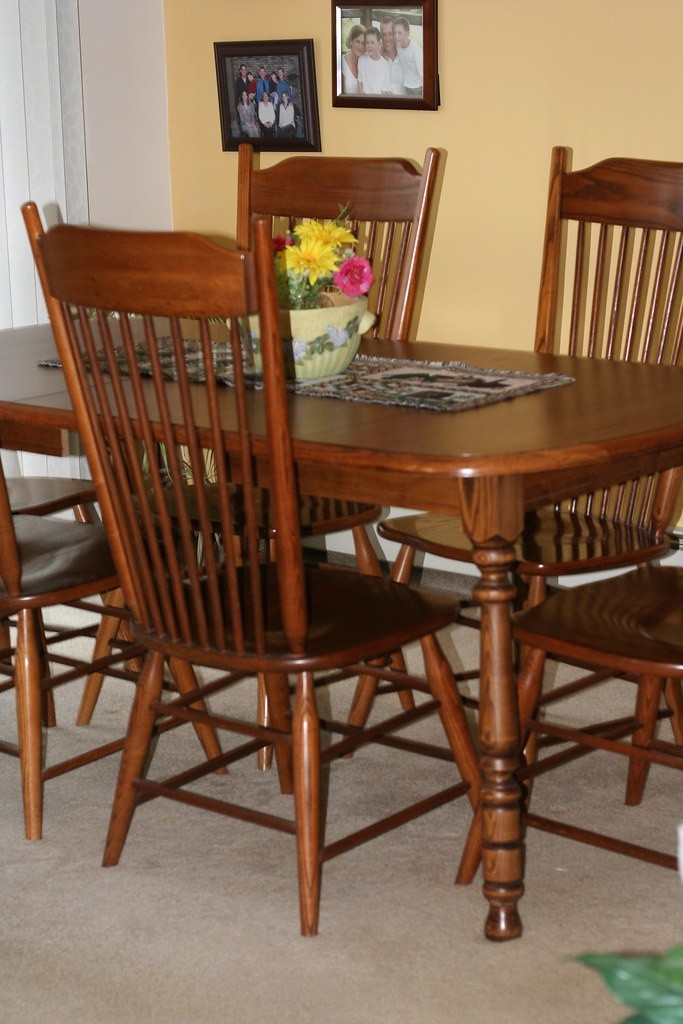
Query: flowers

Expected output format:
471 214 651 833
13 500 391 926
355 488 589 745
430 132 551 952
271 200 373 311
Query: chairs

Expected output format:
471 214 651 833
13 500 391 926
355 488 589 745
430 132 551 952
7 477 144 680
339 145 683 759
0 449 229 842
453 564 683 889
68 141 441 773
21 200 485 937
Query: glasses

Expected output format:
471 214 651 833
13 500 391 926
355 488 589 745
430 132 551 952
381 32 394 37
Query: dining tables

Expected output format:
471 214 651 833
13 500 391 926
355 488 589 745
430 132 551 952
0 316 683 942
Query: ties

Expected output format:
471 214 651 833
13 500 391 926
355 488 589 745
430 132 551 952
263 80 266 91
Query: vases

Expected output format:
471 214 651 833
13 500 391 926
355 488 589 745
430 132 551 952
226 292 377 384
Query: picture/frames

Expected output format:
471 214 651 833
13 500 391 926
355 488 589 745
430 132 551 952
329 1 440 111
213 38 322 153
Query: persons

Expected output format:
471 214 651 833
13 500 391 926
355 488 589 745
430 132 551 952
342 16 424 97
234 64 296 138
